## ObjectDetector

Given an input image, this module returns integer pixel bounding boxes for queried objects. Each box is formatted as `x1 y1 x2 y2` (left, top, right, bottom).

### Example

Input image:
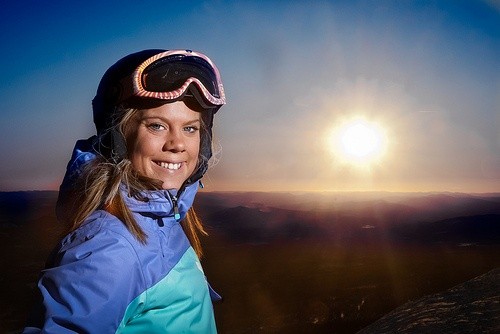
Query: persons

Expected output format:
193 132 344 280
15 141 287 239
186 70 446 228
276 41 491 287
21 50 223 334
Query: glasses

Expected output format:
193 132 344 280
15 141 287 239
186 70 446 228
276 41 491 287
121 49 226 114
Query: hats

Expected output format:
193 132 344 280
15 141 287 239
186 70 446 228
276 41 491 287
92 49 216 199
